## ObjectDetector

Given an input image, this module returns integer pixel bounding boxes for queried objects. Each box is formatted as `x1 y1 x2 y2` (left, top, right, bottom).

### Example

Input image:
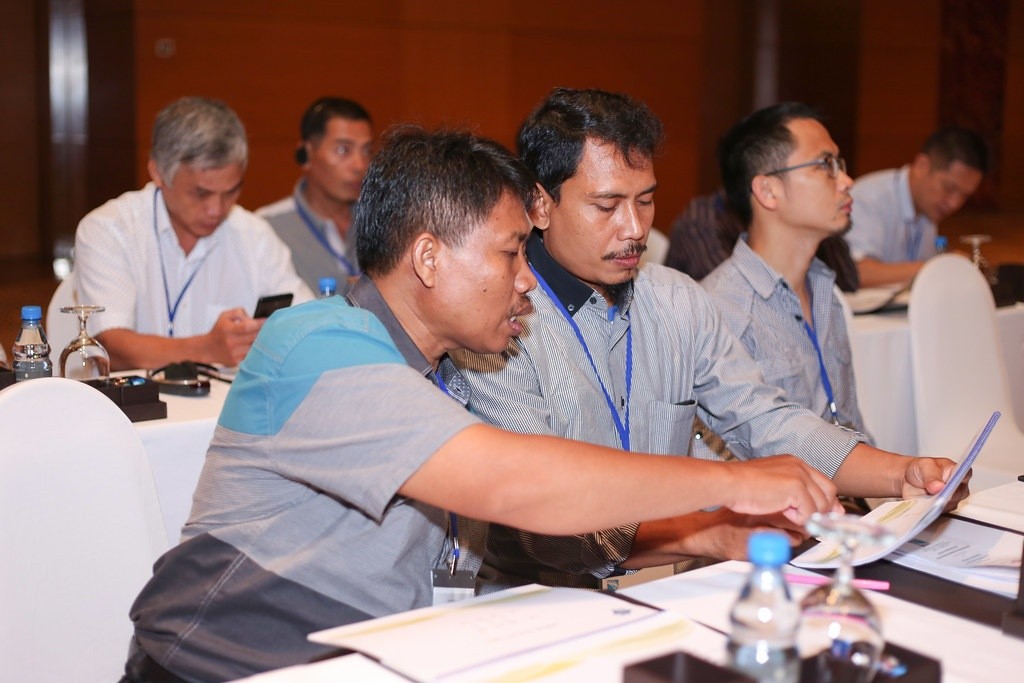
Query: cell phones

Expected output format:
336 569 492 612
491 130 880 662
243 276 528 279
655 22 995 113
254 294 294 320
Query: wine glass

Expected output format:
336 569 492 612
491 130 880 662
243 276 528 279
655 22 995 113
799 511 891 683
58 305 109 380
961 236 995 285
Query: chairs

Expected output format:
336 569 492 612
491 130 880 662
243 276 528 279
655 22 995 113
906 252 1024 495
0 376 167 683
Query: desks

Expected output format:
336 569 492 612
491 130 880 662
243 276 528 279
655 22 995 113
237 479 1024 683
111 283 1024 548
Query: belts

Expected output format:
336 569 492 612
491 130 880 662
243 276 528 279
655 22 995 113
126 635 187 683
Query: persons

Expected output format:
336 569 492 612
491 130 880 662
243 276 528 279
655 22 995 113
663 123 987 291
116 127 841 683
47 98 318 374
695 101 874 516
252 97 378 300
443 85 973 584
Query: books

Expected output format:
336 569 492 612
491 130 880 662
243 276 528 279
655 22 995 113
787 411 998 569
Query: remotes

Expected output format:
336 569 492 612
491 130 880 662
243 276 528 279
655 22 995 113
151 377 209 396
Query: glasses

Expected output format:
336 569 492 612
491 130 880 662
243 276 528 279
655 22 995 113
749 155 847 192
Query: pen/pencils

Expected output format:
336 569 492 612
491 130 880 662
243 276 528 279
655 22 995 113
783 572 892 592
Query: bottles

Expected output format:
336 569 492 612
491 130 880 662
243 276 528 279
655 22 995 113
932 238 948 255
727 530 802 683
318 278 337 299
12 305 53 382
